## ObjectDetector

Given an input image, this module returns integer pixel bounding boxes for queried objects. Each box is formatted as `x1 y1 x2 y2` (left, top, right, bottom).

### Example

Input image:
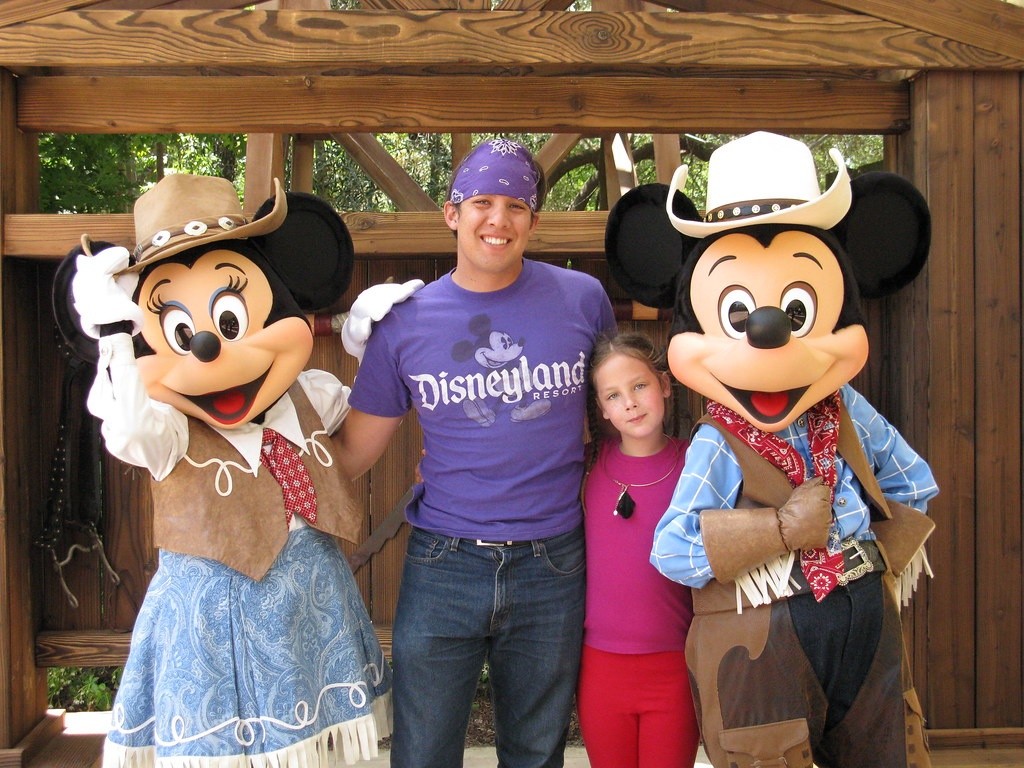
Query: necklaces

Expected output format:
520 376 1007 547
603 431 680 515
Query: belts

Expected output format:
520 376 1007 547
472 536 528 547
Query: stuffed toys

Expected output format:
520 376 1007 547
51 175 426 768
604 132 939 768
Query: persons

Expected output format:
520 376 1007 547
577 333 703 768
314 139 618 768
